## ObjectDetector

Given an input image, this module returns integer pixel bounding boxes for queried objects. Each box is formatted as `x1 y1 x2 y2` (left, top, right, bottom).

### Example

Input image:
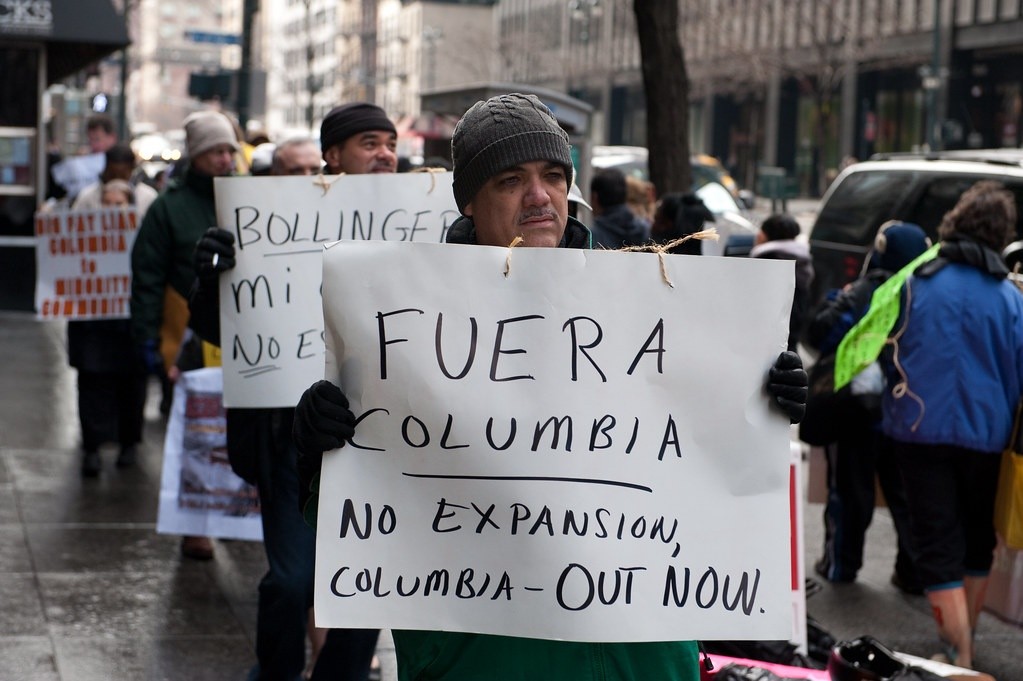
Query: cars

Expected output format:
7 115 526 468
415 85 762 258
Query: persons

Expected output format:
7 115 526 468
873 178 1023 666
798 222 936 596
46 93 810 681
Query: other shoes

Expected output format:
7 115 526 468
179 534 214 563
816 562 856 585
892 575 927 598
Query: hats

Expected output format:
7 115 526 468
319 103 397 161
873 222 931 273
105 145 136 166
450 93 573 220
762 214 800 242
648 194 715 256
183 109 241 160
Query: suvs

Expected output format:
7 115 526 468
802 158 1023 313
868 148 1023 168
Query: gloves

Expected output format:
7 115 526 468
191 228 236 281
764 351 808 425
294 379 356 451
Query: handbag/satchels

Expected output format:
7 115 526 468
798 297 869 446
